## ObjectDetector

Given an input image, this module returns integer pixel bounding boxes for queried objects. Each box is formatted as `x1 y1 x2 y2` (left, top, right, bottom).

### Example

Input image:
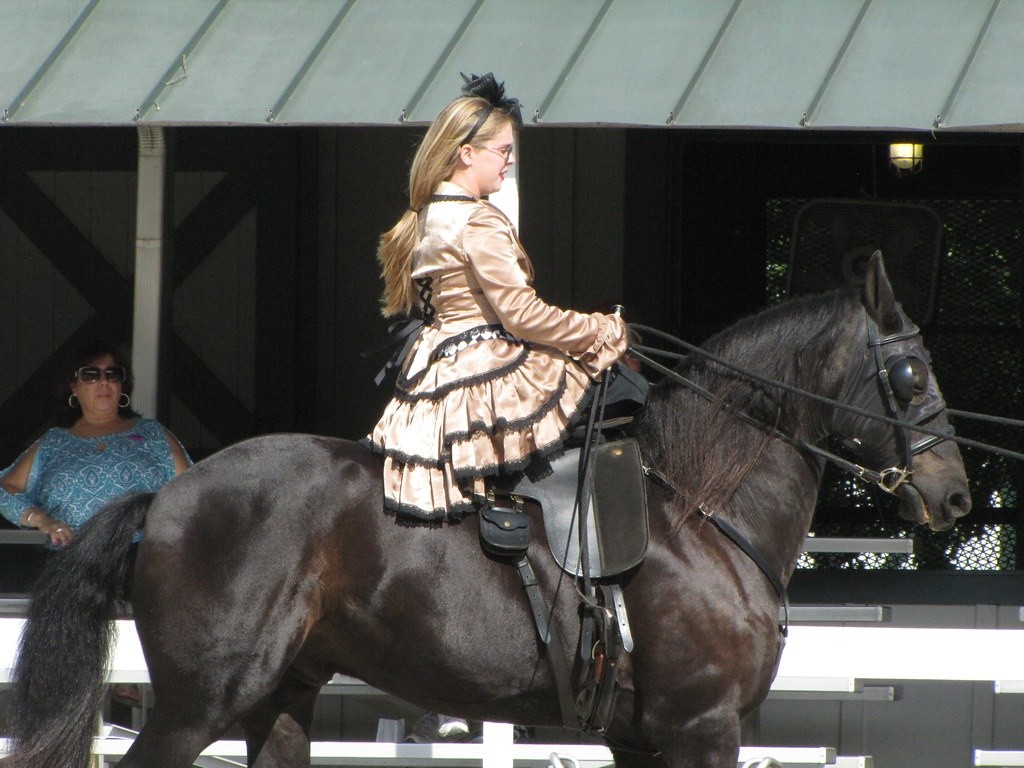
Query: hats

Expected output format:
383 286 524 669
629 328 643 343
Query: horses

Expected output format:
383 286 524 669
1 250 971 768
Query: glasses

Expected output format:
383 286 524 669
478 144 513 161
624 351 634 359
72 366 125 384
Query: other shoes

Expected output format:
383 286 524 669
108 683 138 707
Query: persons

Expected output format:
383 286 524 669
368 72 644 743
0 345 195 710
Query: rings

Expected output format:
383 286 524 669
56 528 65 533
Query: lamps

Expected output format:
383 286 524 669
887 143 925 176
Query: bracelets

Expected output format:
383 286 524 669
27 508 42 529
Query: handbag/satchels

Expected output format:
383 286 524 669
478 509 530 561
